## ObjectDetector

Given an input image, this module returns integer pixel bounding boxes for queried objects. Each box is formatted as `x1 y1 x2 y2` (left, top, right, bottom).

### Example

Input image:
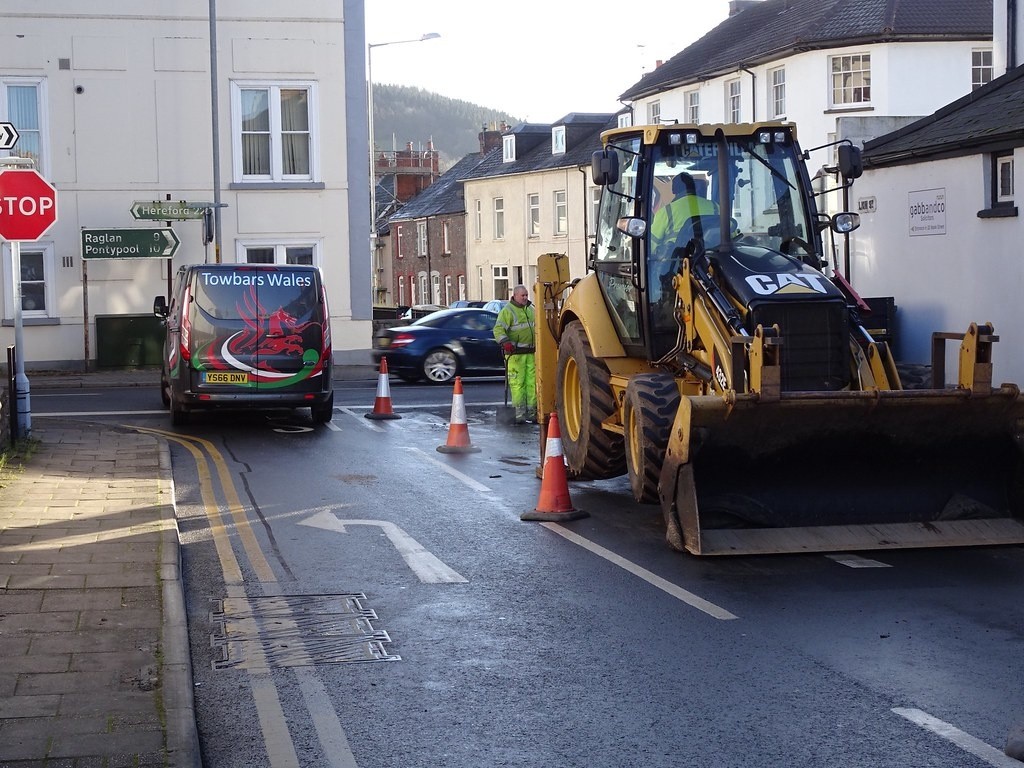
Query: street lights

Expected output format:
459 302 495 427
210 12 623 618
366 32 443 302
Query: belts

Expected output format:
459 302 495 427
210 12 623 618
502 346 536 354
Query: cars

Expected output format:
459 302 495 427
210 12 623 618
481 299 511 313
399 304 449 320
370 307 510 386
449 300 487 309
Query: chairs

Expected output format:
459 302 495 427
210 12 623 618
665 215 738 273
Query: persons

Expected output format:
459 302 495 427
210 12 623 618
650 171 722 259
493 285 538 424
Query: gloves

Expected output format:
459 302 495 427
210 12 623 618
505 342 514 352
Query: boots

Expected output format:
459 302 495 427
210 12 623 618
527 408 540 424
514 406 526 424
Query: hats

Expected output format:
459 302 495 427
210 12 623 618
671 173 695 193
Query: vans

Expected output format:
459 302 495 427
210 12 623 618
152 262 335 425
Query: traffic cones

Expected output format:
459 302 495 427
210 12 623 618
519 410 591 523
435 375 483 454
364 356 403 421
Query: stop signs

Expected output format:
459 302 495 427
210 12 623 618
0 168 58 243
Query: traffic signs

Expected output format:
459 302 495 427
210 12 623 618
80 227 181 262
129 199 206 222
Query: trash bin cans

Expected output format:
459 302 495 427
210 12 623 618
95 314 168 371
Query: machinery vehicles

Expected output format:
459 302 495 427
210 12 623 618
531 114 1024 559
